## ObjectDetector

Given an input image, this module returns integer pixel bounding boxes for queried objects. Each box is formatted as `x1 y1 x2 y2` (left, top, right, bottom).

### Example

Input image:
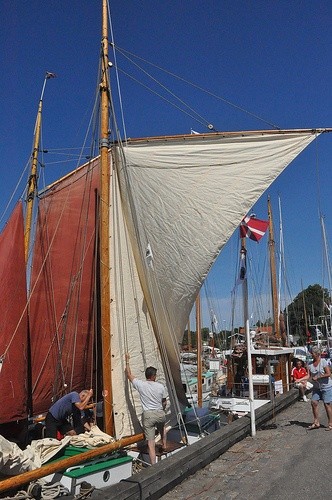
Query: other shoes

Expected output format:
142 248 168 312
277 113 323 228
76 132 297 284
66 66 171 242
309 422 320 430
162 446 168 453
325 423 332 431
300 395 309 402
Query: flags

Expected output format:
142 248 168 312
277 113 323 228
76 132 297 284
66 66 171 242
240 214 269 242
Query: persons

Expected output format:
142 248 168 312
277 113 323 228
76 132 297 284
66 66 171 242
43 388 93 439
125 352 169 466
306 346 332 431
291 360 308 402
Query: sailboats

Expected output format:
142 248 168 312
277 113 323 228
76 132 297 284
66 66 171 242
0 1 332 500
